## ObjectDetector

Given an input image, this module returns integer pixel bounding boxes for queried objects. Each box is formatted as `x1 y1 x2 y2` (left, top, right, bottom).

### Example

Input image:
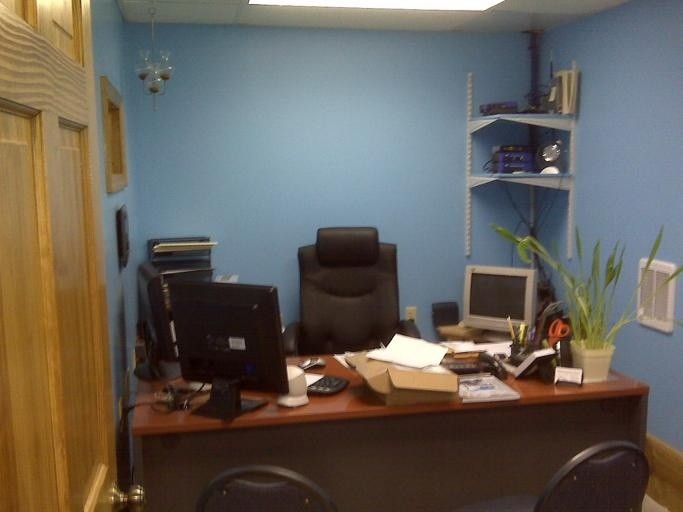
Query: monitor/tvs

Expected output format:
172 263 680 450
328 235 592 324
463 264 538 343
166 277 289 420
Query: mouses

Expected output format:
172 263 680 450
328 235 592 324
298 355 325 372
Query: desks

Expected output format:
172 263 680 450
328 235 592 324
132 354 651 509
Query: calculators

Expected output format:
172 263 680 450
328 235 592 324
308 377 348 395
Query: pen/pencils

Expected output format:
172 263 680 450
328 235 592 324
507 315 536 348
541 338 557 372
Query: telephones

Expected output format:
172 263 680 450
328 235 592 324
446 353 501 379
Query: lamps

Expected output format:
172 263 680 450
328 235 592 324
135 5 176 112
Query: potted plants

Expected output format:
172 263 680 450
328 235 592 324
491 221 683 385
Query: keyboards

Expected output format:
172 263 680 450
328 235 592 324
306 375 349 395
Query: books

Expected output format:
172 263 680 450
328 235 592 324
547 59 578 116
364 332 521 404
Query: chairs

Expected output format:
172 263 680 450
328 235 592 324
534 439 651 511
281 227 422 356
196 465 337 512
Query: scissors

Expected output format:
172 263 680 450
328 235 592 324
548 319 570 348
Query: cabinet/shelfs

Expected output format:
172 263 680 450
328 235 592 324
467 112 575 192
